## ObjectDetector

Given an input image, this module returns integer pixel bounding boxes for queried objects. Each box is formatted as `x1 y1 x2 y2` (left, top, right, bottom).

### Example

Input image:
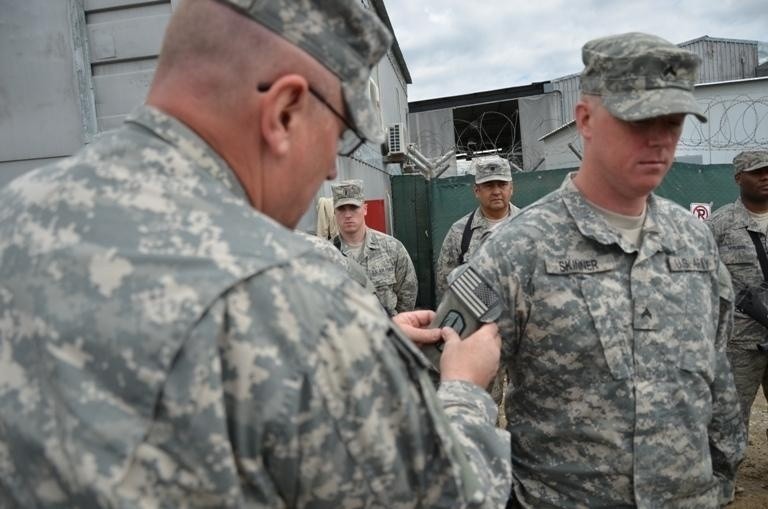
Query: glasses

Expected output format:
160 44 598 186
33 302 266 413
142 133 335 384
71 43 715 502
255 74 367 157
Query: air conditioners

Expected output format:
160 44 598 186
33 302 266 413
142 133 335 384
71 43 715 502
387 122 409 157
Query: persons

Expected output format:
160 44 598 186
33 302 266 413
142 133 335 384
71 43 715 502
421 32 748 509
0 1 513 509
327 179 418 318
435 156 520 432
701 152 768 435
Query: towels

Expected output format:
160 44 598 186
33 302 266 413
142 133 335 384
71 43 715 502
316 196 340 241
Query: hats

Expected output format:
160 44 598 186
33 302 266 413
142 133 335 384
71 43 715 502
733 150 768 177
475 155 512 185
577 31 708 126
331 179 364 209
217 0 395 145
